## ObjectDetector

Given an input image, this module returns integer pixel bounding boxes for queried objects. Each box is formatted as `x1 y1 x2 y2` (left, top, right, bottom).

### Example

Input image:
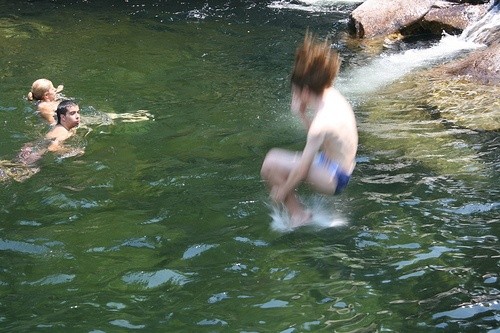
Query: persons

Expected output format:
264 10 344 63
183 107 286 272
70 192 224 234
26 78 81 140
258 27 359 230
